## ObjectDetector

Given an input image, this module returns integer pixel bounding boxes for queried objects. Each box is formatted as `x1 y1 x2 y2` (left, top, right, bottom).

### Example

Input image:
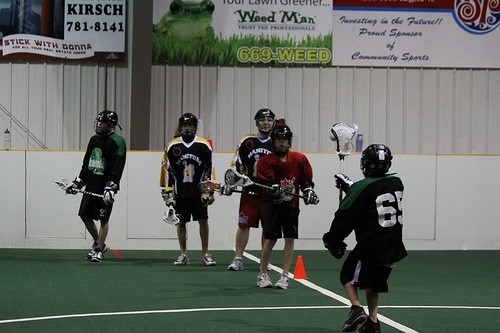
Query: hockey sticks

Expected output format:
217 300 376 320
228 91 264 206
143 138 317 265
162 203 181 224
51 180 115 202
198 177 261 197
224 167 307 199
330 121 359 208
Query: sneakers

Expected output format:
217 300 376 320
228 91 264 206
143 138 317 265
260 262 272 270
201 255 216 265
227 258 243 271
358 316 381 333
257 273 273 288
85 243 107 262
342 305 366 332
275 275 289 290
174 254 191 265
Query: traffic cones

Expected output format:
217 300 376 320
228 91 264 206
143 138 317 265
293 255 308 280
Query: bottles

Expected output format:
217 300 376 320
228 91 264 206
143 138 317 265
4 129 11 149
356 133 363 152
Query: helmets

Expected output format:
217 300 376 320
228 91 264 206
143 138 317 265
270 124 292 153
360 144 392 176
254 109 274 134
92 110 118 135
179 113 197 142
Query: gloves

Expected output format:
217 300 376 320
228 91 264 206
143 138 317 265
201 191 214 207
103 181 118 206
265 183 285 204
323 232 348 259
334 173 353 193
301 183 319 205
222 170 237 195
161 188 176 207
66 177 86 195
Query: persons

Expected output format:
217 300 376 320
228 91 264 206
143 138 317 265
254 124 319 289
221 108 276 271
323 144 408 333
160 112 216 266
65 111 126 261
